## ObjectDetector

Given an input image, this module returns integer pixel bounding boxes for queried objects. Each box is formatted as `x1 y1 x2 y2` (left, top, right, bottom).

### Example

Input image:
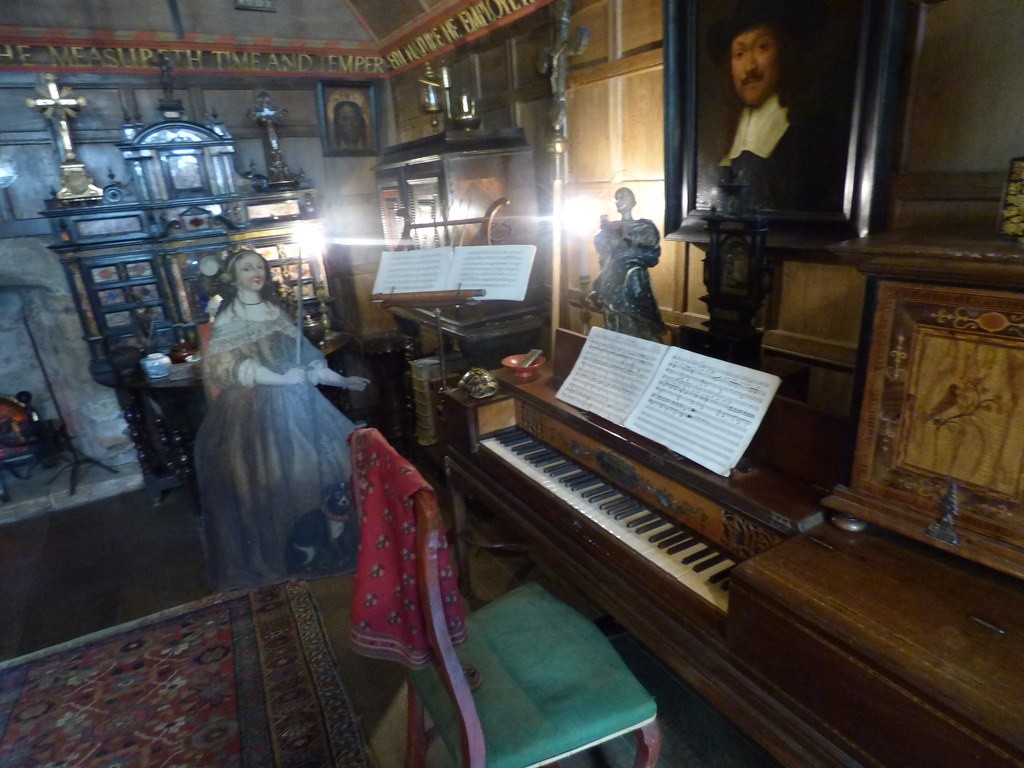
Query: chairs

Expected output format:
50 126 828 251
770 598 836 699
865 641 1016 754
347 425 665 768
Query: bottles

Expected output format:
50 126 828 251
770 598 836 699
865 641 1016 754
139 353 171 378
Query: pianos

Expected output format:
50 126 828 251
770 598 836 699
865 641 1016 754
442 328 1024 768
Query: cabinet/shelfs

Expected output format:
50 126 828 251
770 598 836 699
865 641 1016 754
388 297 549 394
89 323 360 515
437 370 1024 768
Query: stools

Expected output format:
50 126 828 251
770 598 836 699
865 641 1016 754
361 334 416 437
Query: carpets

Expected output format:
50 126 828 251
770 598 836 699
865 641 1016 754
1 574 379 768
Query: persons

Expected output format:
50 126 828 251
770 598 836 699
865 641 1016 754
707 5 830 208
185 243 392 590
332 100 374 155
590 187 661 323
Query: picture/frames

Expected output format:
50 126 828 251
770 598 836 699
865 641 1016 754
662 0 917 254
316 79 380 157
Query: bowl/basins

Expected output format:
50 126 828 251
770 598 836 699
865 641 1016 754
500 354 547 378
